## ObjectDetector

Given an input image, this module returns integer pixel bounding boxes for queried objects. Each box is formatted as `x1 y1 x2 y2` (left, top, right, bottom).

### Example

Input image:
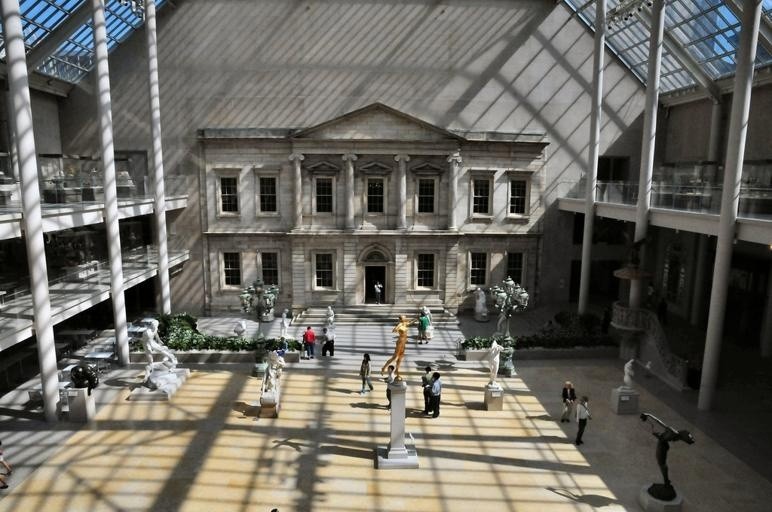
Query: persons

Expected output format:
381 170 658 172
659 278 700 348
419 312 428 344
619 359 634 389
0 476 8 490
278 337 288 355
359 354 373 393
473 288 486 312
381 315 418 377
637 412 696 490
422 366 434 414
321 328 336 356
488 340 504 385
561 381 576 423
374 280 383 304
142 320 174 383
386 366 395 409
427 372 441 417
0 436 14 474
304 327 316 360
327 306 334 326
280 309 289 337
575 396 590 445
